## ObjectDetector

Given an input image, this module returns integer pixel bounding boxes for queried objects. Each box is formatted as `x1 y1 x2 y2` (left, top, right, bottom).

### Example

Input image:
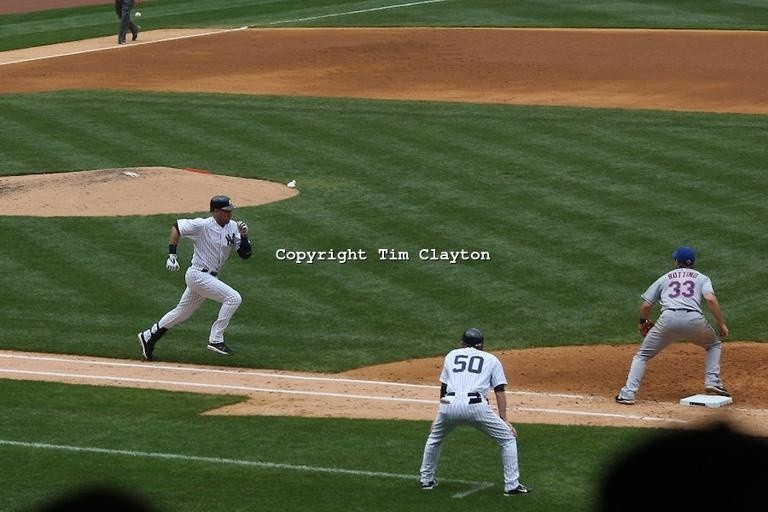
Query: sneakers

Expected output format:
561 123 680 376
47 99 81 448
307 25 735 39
118 39 127 45
206 342 232 357
704 382 731 398
615 394 636 405
421 479 440 490
132 24 141 41
505 484 530 496
137 328 154 360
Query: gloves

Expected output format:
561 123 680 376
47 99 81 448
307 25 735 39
166 252 181 272
236 219 249 235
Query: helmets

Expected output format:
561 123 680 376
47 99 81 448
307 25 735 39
463 328 484 350
209 195 236 214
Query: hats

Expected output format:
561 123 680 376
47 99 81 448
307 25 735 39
673 247 696 265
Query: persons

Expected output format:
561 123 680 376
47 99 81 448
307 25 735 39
614 247 730 404
137 196 252 361
115 0 141 44
419 326 534 496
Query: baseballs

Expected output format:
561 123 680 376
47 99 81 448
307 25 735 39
134 11 142 19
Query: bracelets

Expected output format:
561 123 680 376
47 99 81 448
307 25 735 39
639 318 647 324
167 244 177 254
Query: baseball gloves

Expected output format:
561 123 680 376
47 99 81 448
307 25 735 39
639 320 654 336
